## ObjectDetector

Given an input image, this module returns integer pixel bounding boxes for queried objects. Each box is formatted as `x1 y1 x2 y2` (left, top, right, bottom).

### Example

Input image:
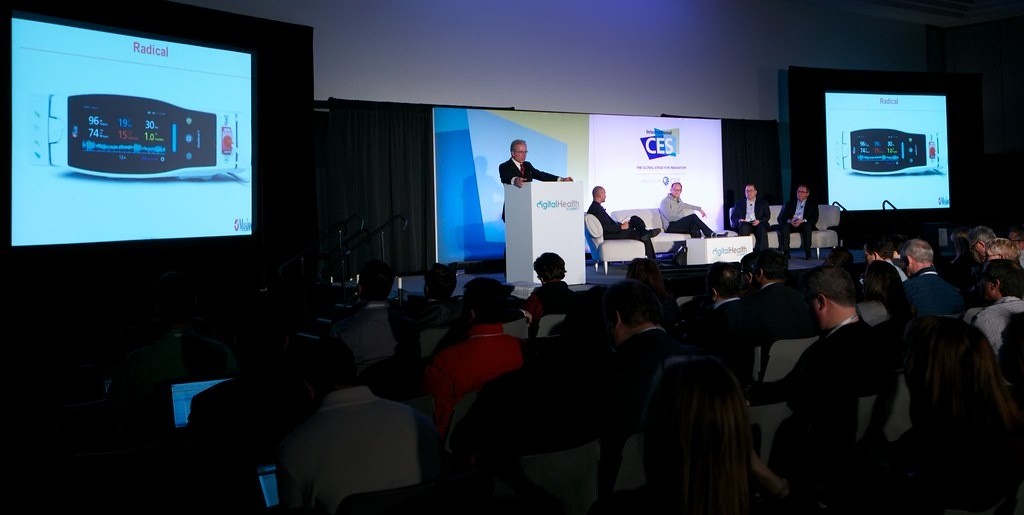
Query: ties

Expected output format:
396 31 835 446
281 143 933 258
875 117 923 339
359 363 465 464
520 164 524 177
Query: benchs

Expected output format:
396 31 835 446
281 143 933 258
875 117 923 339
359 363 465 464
729 205 840 259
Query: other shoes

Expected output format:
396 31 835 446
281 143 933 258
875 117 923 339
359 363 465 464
717 232 728 237
640 228 661 241
806 256 813 260
785 255 791 259
657 262 662 267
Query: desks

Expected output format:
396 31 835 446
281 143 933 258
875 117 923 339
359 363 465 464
679 236 752 267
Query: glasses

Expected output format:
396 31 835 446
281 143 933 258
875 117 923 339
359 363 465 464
515 150 528 154
673 189 682 192
986 253 1003 259
970 241 978 251
797 191 808 194
746 190 753 192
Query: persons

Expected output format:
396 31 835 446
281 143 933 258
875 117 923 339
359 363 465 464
0 225 1024 515
776 185 819 260
659 182 728 238
586 186 661 263
499 139 573 278
731 184 770 250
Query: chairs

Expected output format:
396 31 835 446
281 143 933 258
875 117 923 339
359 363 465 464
585 213 647 275
501 318 529 339
747 401 793 472
645 209 689 255
334 479 449 515
751 335 820 383
882 371 920 443
537 314 566 349
520 436 605 515
402 394 481 453
420 328 448 356
